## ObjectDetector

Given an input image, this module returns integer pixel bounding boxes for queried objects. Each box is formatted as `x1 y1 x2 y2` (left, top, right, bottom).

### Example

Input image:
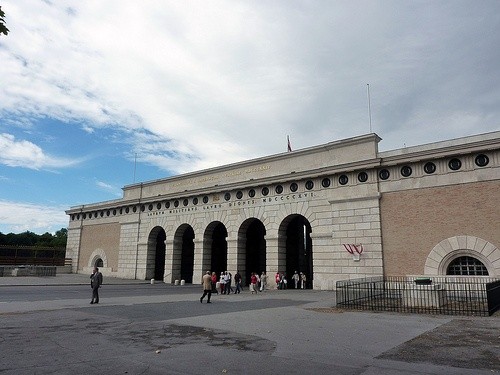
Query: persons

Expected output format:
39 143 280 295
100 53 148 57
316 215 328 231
250 272 258 295
219 271 227 294
254 272 260 292
299 271 307 290
234 269 244 294
291 270 300 289
223 271 230 296
259 271 270 292
199 270 213 304
227 272 235 293
279 272 287 290
210 272 219 295
276 273 281 290
88 267 103 304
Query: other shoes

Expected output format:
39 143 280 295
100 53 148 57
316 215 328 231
200 298 202 303
207 301 211 303
227 292 230 295
90 302 99 304
234 291 240 294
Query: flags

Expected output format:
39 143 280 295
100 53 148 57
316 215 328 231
287 137 292 153
